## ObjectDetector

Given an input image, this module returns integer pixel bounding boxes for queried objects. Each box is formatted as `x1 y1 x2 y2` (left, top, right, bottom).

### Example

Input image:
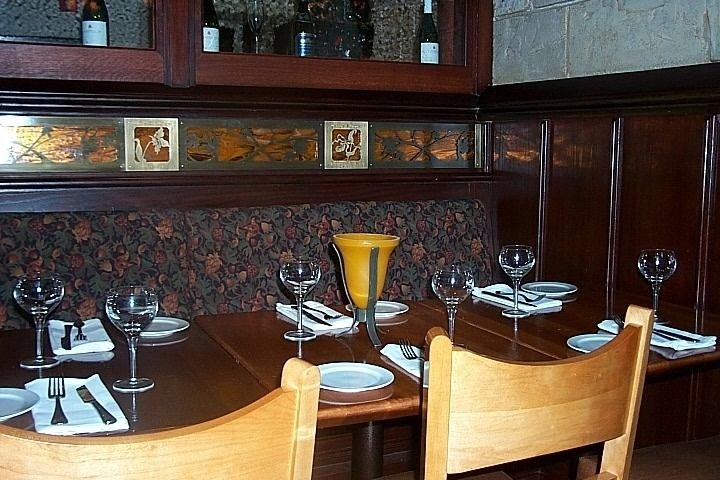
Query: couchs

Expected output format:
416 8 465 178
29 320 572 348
0 199 491 328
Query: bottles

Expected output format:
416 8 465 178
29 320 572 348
293 0 315 58
82 0 109 47
201 0 220 54
419 0 439 63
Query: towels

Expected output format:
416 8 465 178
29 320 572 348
599 317 716 349
473 282 563 312
47 318 116 354
275 300 358 331
27 373 128 435
382 342 426 378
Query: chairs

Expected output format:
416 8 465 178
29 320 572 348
0 357 321 480
377 303 654 480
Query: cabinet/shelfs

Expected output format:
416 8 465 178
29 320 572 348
11 0 478 91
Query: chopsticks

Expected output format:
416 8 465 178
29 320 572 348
482 290 537 308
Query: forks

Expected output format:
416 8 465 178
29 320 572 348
496 290 547 302
48 373 68 425
398 337 428 365
72 318 87 341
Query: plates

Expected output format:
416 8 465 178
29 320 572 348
140 317 191 336
345 300 410 319
2 388 42 421
318 362 396 392
567 334 619 352
522 282 578 297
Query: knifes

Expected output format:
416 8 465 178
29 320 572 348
61 323 73 349
289 306 333 328
77 384 118 425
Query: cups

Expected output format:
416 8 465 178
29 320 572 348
12 274 65 368
280 258 323 340
105 286 156 393
498 245 535 318
636 250 676 324
430 266 475 353
